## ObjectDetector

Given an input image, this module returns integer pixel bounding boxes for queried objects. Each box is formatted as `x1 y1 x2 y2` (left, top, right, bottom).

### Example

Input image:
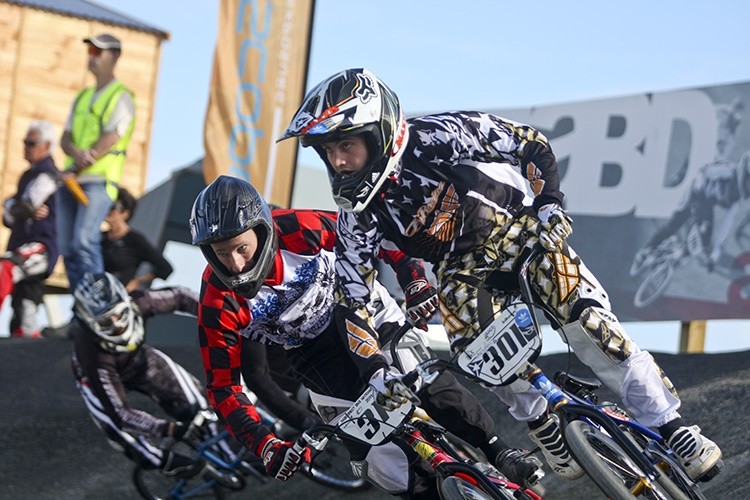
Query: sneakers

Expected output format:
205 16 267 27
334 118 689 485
498 448 543 485
537 421 585 481
666 425 722 480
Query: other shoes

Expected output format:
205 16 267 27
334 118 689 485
43 320 74 341
162 450 206 480
9 326 40 339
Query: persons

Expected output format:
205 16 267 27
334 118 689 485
3 121 60 337
99 187 172 290
272 66 724 479
42 34 136 338
67 272 206 478
636 151 750 271
189 176 543 500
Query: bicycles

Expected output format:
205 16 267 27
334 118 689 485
131 306 546 499
630 211 718 309
376 217 727 500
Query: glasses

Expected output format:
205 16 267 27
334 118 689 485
110 205 116 210
94 304 132 336
89 47 101 55
24 140 34 147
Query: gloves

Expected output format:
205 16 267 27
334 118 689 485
396 261 440 331
172 408 219 447
537 202 573 252
256 434 312 482
366 364 421 413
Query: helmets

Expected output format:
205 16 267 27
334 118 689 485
188 176 277 299
73 270 145 352
279 69 410 213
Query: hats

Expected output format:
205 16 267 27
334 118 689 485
83 34 122 50
117 187 136 221
